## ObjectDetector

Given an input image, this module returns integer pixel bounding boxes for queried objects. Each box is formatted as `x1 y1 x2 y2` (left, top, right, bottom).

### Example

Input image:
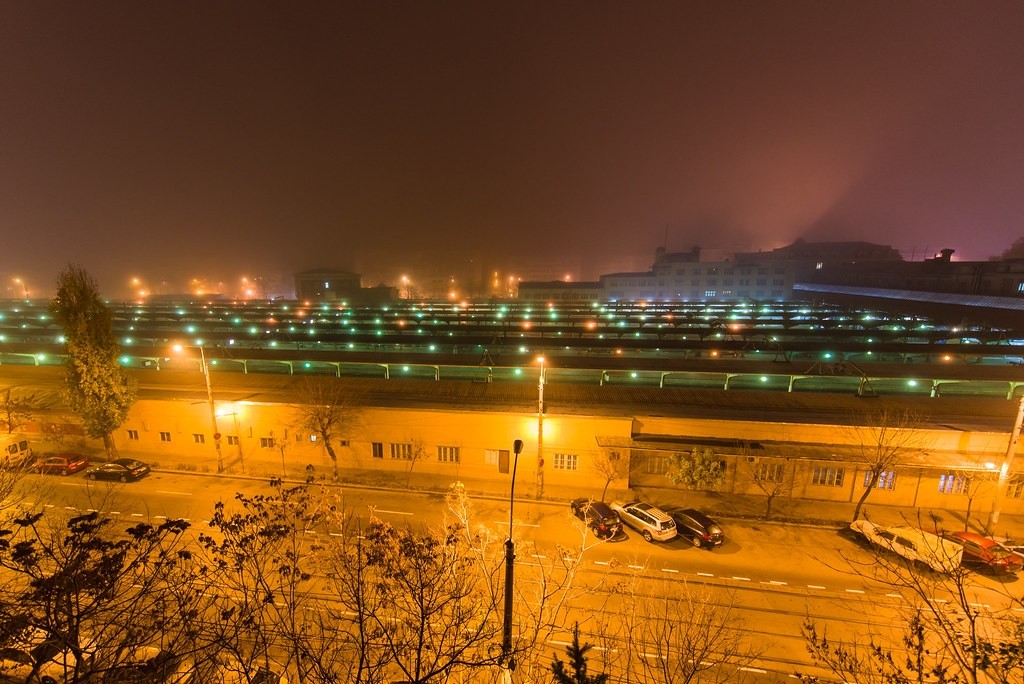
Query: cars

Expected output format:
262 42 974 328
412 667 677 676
660 505 724 548
88 459 150 482
570 497 623 537
995 539 1024 570
31 453 87 475
610 499 677 542
945 531 1022 573
870 524 964 572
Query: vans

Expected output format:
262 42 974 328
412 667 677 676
1 433 33 470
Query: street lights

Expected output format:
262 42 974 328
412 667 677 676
174 344 224 473
2 268 1024 401
502 440 523 653
536 348 546 498
